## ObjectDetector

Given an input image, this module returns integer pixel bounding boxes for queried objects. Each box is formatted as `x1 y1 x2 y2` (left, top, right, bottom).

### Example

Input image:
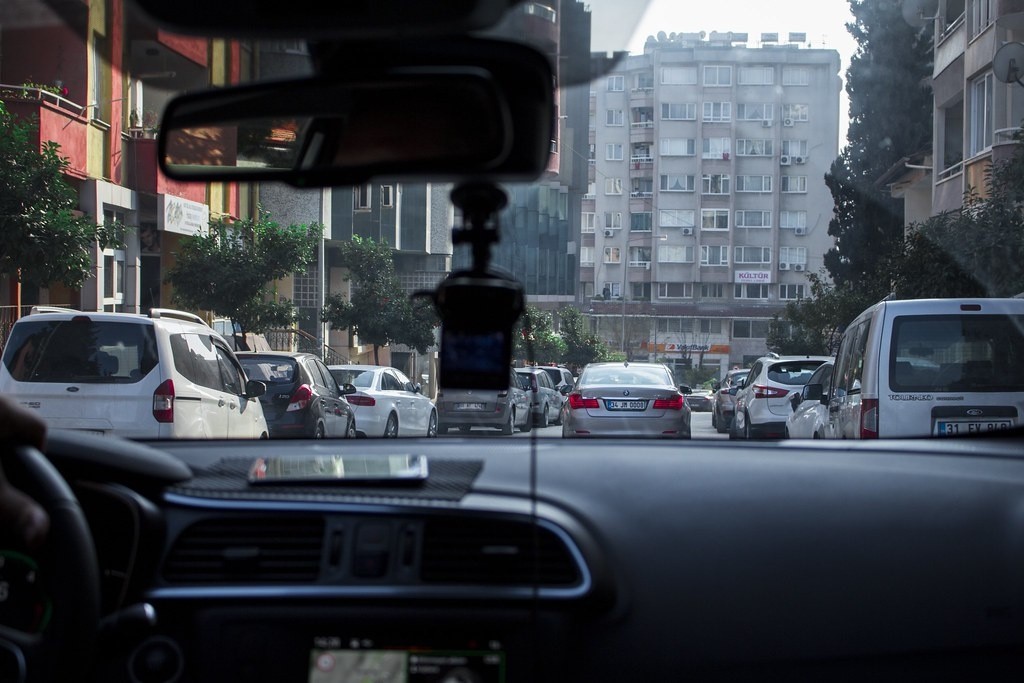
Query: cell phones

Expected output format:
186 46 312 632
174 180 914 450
247 453 429 487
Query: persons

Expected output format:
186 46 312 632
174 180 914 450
323 106 464 170
139 223 160 255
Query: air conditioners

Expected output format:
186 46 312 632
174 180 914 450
783 118 794 126
795 155 805 164
682 228 692 236
780 155 791 166
794 226 806 236
794 264 805 272
604 230 613 237
762 120 772 127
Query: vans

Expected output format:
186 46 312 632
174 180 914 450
0 306 271 444
824 297 1024 438
521 370 567 426
234 351 357 443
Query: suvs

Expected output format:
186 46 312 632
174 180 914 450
726 354 835 440
434 366 534 433
711 368 759 432
535 366 573 395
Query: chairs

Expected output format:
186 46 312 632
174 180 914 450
895 361 995 390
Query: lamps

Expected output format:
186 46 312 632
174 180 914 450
778 262 790 271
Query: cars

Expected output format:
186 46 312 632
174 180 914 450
686 389 716 412
328 364 439 440
784 361 863 439
563 360 695 441
